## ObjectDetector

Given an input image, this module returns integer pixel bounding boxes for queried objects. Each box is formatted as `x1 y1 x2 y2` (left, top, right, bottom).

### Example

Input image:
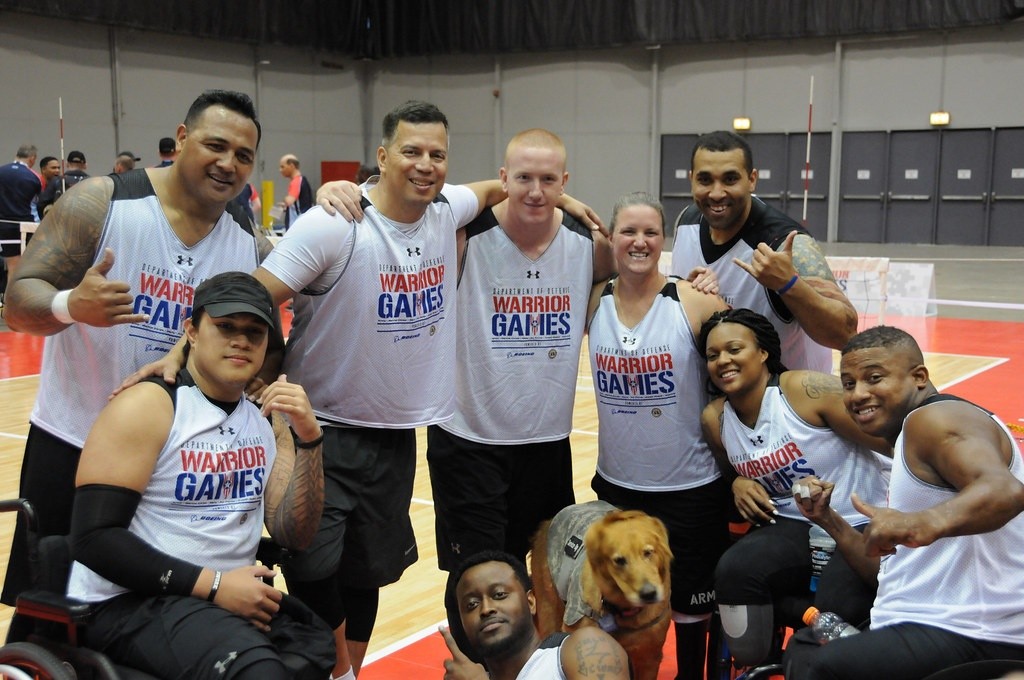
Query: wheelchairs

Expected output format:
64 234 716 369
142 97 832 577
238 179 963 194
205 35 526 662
702 496 809 677
0 497 295 680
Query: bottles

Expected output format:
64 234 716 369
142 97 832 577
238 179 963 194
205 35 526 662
802 606 861 645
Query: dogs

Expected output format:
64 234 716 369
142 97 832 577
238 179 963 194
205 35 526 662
532 500 676 680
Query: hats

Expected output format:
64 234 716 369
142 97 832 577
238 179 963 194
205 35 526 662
159 138 176 154
119 151 141 162
68 151 86 164
194 271 276 329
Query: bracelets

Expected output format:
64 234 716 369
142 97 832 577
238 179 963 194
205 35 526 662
52 288 76 325
776 273 798 298
294 426 324 449
206 571 222 603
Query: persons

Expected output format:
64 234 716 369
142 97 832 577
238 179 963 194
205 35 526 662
0 138 261 314
104 99 609 680
62 271 337 679
700 308 893 680
585 193 734 679
1 88 276 669
439 550 630 679
280 153 312 232
792 325 1024 679
317 126 721 671
668 129 859 374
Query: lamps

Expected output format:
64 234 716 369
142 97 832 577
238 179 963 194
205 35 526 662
258 56 271 65
930 111 951 126
733 116 753 131
